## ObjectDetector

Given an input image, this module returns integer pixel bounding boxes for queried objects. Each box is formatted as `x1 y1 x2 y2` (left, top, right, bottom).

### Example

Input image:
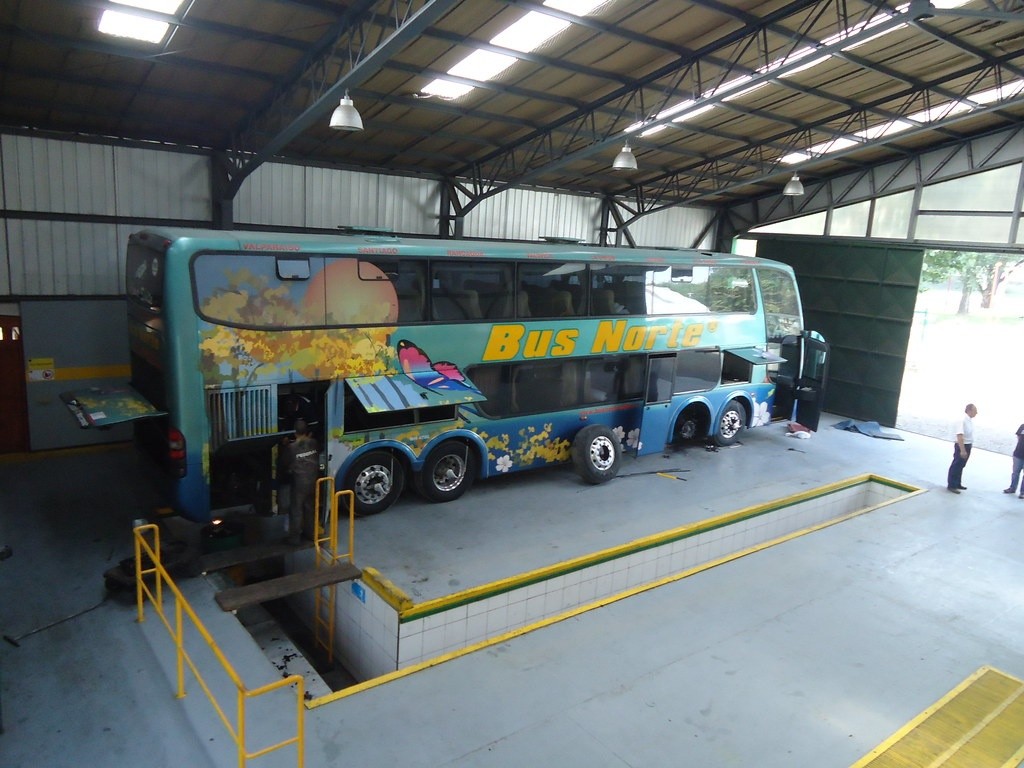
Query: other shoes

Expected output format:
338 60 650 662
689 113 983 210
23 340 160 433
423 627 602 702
1019 493 1024 499
1004 488 1015 492
301 532 314 540
284 537 300 545
948 487 960 494
958 486 968 490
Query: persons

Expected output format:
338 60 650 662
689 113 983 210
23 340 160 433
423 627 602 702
280 420 322 545
948 404 977 494
466 368 480 384
1003 424 1024 498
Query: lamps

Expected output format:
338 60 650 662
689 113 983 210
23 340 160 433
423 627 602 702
613 141 642 170
783 170 804 195
329 89 364 131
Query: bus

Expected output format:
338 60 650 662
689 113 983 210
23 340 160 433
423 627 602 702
61 226 832 527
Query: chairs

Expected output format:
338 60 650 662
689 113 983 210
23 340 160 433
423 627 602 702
385 264 633 414
